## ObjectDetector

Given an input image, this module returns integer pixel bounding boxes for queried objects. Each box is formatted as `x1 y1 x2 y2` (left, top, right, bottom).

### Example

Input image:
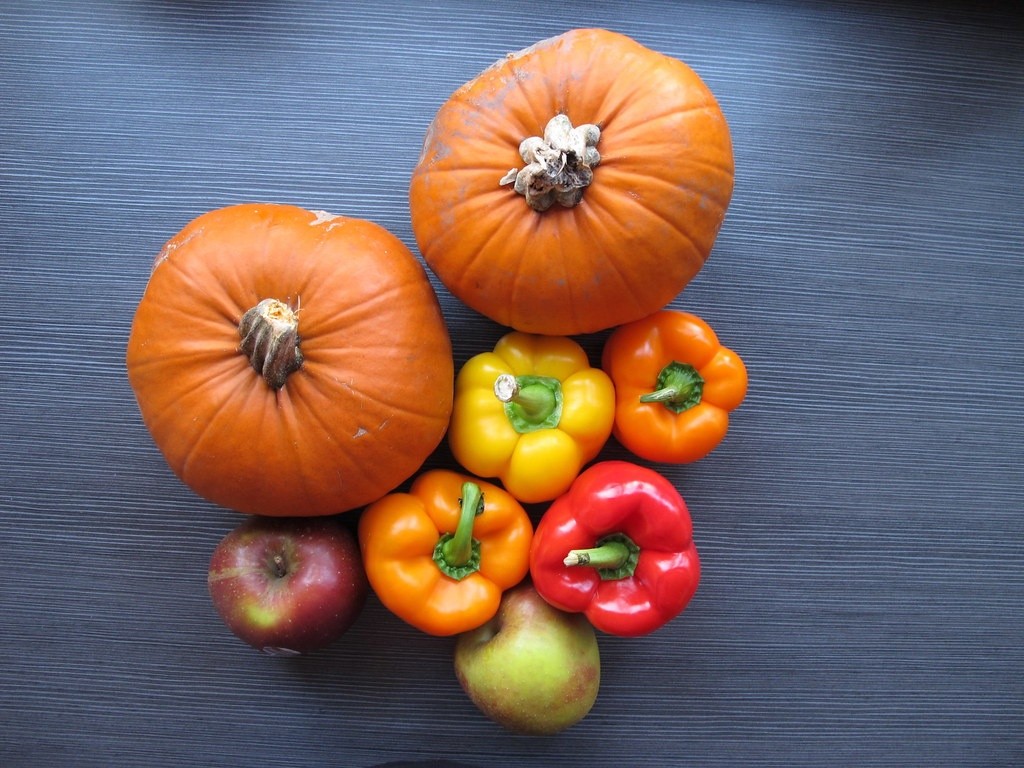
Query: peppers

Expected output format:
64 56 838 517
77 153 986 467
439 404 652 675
354 309 748 637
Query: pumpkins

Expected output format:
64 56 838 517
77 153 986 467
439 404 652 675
408 29 735 336
127 203 455 515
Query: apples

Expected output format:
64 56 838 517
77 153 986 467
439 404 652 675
454 585 600 736
207 515 365 654
